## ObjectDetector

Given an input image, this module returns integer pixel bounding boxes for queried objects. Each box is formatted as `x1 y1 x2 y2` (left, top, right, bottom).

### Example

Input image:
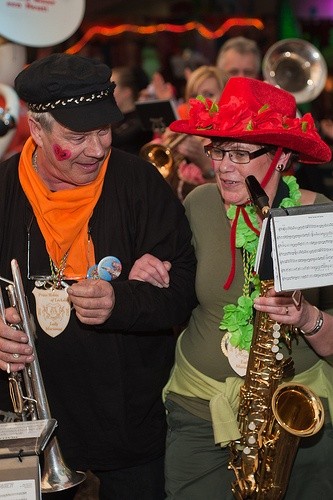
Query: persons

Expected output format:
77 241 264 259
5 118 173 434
101 261 121 279
111 37 333 201
0 53 194 500
128 76 333 500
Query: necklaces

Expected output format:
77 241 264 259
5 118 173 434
32 150 70 338
227 250 255 377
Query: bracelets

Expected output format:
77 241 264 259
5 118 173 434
300 305 323 336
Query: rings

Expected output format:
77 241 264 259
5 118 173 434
286 306 288 315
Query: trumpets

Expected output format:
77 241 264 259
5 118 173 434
262 38 329 104
138 132 192 177
0 257 87 495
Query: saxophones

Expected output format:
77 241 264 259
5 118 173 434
225 174 325 500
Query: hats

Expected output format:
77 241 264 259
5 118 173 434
14 53 123 132
169 76 332 165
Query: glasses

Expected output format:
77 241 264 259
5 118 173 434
27 229 91 280
203 143 274 164
